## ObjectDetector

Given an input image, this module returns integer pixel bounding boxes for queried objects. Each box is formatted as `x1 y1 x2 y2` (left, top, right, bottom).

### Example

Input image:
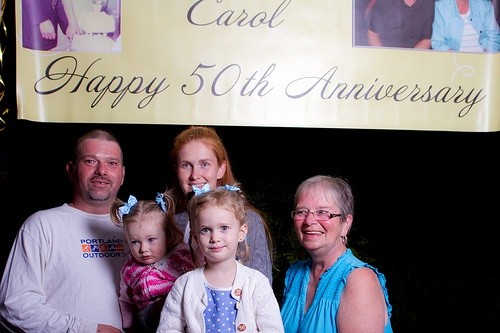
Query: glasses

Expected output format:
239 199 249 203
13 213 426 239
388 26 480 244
290 211 342 220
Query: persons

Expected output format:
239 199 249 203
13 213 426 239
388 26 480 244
280 176 392 333
22 0 120 52
109 191 206 333
364 0 500 53
170 128 273 288
0 124 127 333
157 183 284 333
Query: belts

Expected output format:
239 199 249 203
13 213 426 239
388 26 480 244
78 32 114 36
